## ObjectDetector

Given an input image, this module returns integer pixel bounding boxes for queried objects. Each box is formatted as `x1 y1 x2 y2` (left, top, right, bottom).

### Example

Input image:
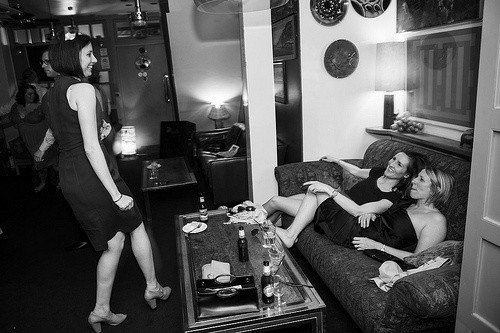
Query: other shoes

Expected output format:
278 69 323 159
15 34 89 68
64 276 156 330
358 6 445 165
33 182 46 192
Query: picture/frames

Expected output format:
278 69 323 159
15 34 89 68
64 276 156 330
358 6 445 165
271 13 297 62
274 62 288 104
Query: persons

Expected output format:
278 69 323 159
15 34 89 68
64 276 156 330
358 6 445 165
42 34 170 333
0 51 88 248
261 138 455 267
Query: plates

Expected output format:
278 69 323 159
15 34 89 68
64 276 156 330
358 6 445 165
182 221 207 234
310 0 391 78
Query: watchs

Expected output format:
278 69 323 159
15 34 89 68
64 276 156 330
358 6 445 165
330 190 338 198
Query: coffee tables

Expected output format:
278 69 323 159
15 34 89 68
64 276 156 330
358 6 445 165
175 204 327 333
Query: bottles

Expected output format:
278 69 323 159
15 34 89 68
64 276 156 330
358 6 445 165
200 193 209 222
261 261 275 304
238 226 250 262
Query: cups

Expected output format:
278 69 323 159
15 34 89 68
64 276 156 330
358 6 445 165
260 224 276 248
268 247 284 269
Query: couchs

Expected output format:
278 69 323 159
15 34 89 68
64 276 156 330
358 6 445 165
275 139 470 333
195 122 289 207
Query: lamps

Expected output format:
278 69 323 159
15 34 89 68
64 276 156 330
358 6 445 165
46 0 79 45
131 0 148 26
121 126 136 158
208 102 231 129
375 41 407 128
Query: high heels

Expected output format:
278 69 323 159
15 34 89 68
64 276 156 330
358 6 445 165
144 286 171 310
88 311 128 333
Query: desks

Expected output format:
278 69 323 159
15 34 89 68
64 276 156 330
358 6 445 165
142 156 199 223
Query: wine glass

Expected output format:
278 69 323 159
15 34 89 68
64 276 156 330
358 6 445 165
269 274 288 315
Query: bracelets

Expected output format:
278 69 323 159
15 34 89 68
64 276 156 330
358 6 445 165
338 159 341 164
114 194 123 202
381 244 385 252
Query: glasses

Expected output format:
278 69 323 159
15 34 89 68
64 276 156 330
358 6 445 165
39 59 50 65
